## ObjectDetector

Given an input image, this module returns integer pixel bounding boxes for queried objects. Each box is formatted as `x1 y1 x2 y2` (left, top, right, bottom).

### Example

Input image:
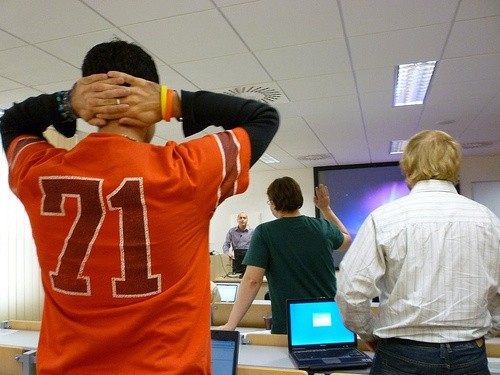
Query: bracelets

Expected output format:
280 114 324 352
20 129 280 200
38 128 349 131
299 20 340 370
56 90 80 122
165 89 174 122
160 85 167 120
176 89 183 122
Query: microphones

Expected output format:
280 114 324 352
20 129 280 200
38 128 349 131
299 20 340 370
240 235 242 237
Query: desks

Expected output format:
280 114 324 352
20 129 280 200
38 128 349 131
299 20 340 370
1 278 500 374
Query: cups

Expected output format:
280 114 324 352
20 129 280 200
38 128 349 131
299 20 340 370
264 316 272 330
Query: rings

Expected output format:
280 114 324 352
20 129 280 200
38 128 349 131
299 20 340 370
117 98 120 105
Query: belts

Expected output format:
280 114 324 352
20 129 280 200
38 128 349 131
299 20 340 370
386 337 467 347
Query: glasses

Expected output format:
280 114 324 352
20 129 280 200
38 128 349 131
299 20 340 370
267 199 273 205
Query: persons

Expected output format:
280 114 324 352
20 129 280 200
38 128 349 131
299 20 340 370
335 131 500 375
223 212 254 279
210 280 222 328
210 176 352 335
0 35 280 375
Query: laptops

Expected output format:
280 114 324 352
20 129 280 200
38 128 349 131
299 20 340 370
286 297 374 371
214 283 240 303
210 330 239 375
